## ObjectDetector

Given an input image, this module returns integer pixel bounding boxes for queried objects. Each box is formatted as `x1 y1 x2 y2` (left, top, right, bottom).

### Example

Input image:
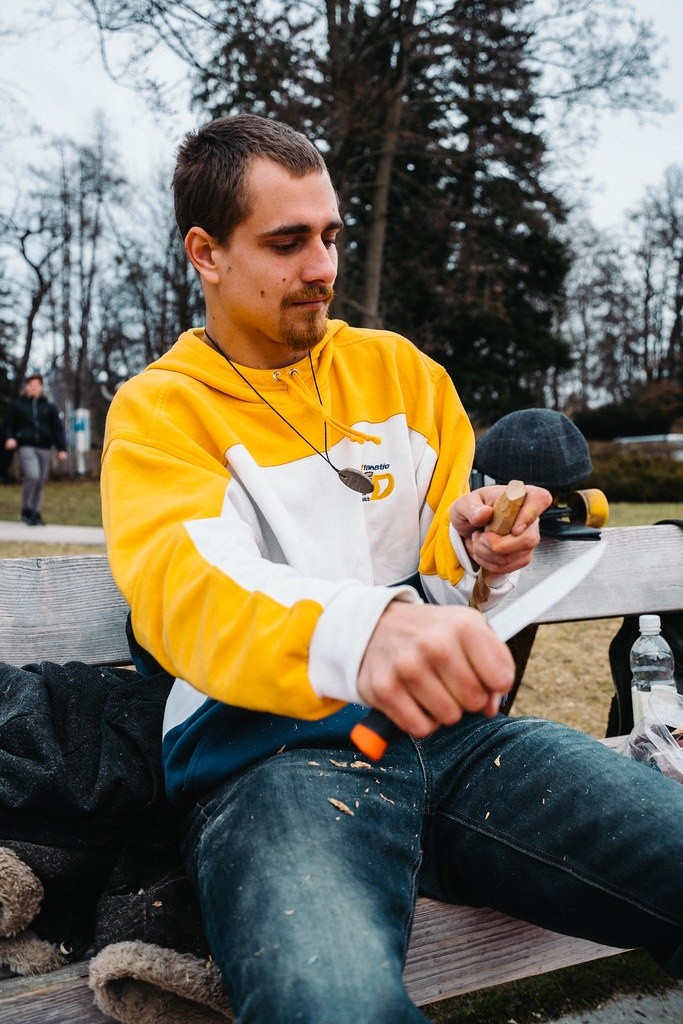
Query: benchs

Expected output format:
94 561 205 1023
0 524 683 1024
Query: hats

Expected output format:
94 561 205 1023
474 408 593 488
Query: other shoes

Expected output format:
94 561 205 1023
35 513 45 526
19 509 36 525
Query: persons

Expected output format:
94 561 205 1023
100 114 683 1024
6 377 69 527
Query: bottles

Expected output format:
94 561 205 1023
630 614 677 727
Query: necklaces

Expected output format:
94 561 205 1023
204 327 374 494
